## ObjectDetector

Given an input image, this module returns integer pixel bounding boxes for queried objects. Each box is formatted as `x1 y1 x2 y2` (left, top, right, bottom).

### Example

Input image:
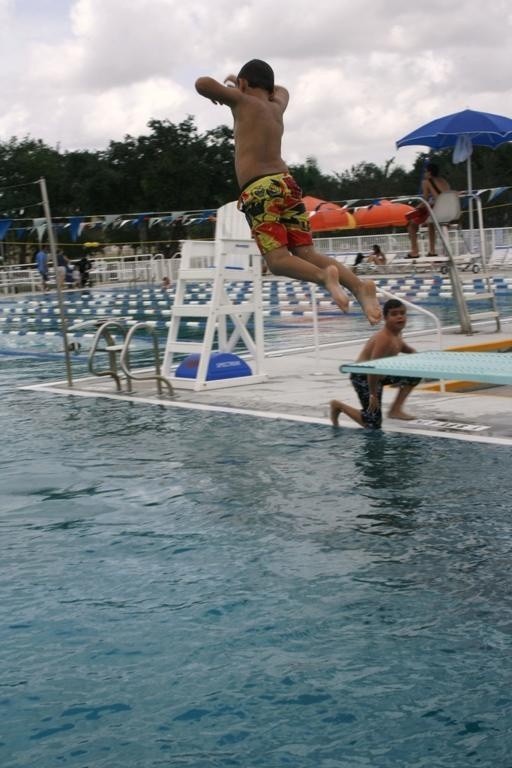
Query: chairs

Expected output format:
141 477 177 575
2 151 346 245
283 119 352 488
158 201 269 393
419 190 460 258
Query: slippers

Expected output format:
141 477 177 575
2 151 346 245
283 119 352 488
404 254 419 259
425 252 437 257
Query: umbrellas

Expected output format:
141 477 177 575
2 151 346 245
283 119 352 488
395 108 511 256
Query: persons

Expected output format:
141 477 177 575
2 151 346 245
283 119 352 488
402 162 450 259
367 244 386 266
57 248 75 291
329 298 423 429
161 276 171 289
36 244 52 291
78 257 92 287
353 252 380 276
195 58 383 326
